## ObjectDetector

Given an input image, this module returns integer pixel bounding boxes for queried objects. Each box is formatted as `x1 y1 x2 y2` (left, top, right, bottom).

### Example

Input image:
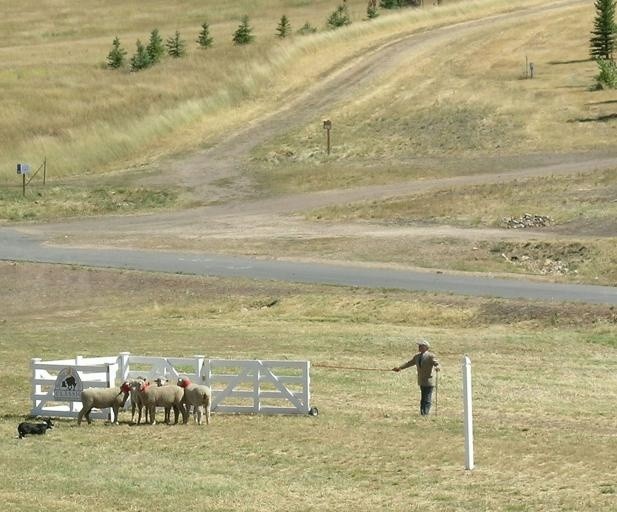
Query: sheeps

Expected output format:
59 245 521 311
77 381 132 426
128 374 212 426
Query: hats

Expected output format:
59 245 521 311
415 340 431 349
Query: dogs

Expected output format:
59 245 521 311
18 417 54 439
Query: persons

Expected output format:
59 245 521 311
393 340 440 415
